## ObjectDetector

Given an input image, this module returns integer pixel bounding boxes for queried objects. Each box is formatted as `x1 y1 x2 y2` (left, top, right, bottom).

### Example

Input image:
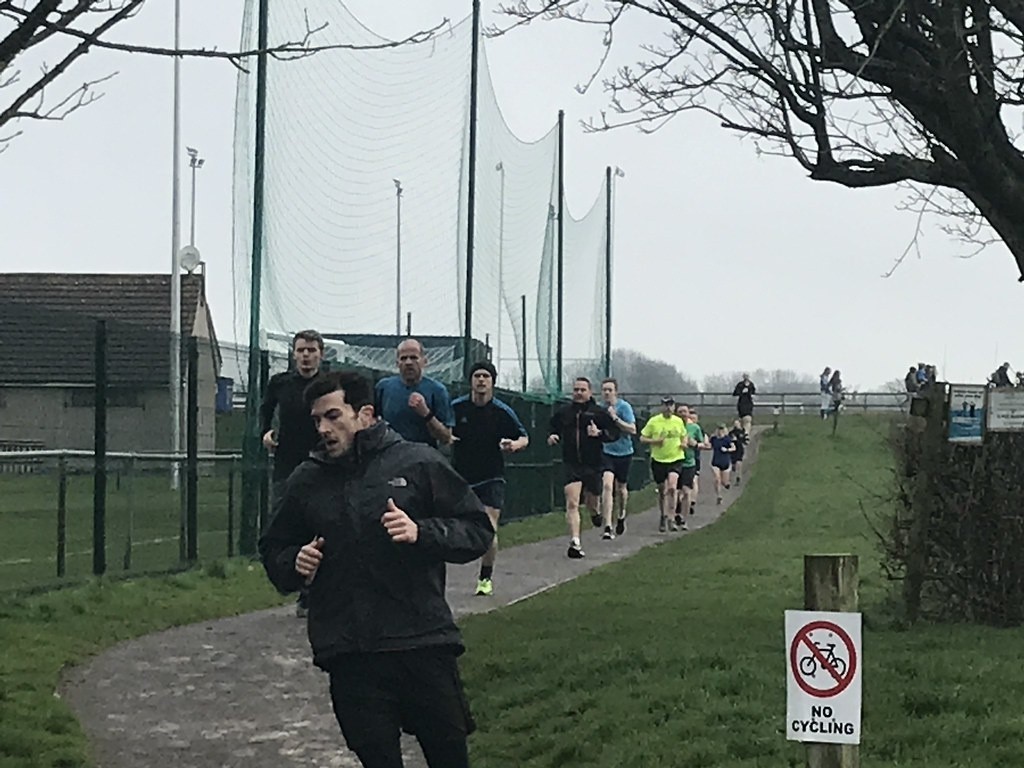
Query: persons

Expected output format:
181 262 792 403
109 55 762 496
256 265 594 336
727 415 751 486
706 423 736 504
820 367 832 418
898 363 936 407
595 379 636 535
826 370 842 415
258 370 496 768
732 373 756 442
674 411 708 515
256 331 342 618
640 395 689 533
440 362 529 596
671 404 704 531
546 377 620 559
373 339 456 453
987 363 1024 390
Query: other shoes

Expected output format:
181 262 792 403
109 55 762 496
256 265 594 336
716 496 722 504
690 501 696 515
724 483 730 489
296 585 311 616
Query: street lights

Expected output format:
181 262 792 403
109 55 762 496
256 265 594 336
187 145 206 246
393 178 402 335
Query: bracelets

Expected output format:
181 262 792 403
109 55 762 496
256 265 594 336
425 409 434 422
613 417 619 423
695 442 698 448
727 448 728 451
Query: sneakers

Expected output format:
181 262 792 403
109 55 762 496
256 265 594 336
600 526 616 540
591 510 603 527
659 515 667 532
674 514 683 526
668 519 678 532
474 579 493 595
567 541 586 559
681 524 688 530
614 518 627 536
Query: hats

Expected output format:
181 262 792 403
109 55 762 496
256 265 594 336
469 361 497 383
661 396 675 403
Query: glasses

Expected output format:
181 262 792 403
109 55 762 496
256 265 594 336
574 387 589 392
